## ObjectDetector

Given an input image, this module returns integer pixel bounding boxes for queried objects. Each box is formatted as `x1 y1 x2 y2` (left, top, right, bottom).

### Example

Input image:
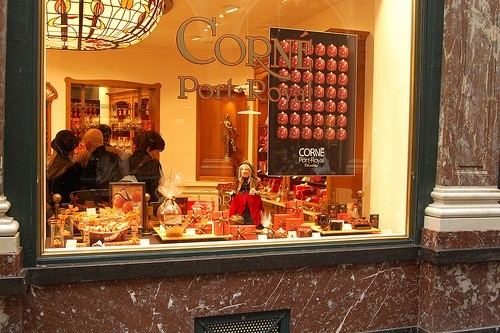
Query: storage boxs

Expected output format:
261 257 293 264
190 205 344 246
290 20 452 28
231 225 257 239
195 224 211 234
272 199 306 229
213 213 229 236
338 214 351 223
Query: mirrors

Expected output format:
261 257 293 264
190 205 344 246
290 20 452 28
63 76 162 159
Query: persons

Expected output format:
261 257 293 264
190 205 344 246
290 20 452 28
122 127 164 203
130 134 142 153
222 114 237 156
77 124 130 177
85 129 123 189
47 130 83 210
228 159 264 227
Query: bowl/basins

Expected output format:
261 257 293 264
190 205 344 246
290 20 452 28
166 225 184 237
330 220 342 230
80 227 130 243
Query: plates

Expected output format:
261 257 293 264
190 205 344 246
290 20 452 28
309 226 380 234
154 227 234 241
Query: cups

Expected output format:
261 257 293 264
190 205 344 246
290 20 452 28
51 219 65 248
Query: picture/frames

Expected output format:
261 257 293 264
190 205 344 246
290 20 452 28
109 182 148 230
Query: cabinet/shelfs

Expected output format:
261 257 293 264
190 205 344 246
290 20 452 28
70 89 151 151
252 27 371 217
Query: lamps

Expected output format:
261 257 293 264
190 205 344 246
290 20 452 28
44 0 174 50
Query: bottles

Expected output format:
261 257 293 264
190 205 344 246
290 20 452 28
70 101 131 143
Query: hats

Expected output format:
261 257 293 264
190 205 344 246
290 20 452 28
50 130 81 153
